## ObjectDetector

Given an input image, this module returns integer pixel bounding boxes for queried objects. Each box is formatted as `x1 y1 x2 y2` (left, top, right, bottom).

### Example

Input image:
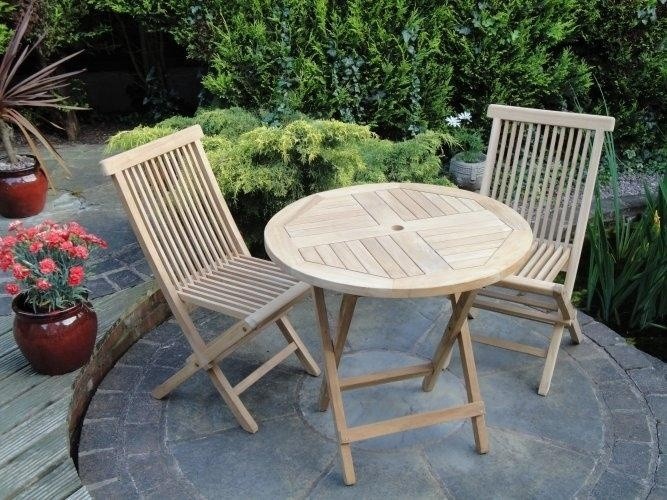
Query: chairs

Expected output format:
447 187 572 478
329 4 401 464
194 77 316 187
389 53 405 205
438 103 617 396
96 123 321 435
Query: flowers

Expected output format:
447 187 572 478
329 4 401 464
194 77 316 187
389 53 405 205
0 218 108 314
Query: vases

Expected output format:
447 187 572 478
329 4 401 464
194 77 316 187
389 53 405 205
11 288 98 375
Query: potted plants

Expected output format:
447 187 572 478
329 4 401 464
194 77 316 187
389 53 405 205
0 0 95 217
444 110 488 192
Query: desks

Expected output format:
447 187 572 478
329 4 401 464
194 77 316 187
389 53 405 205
261 183 535 487
